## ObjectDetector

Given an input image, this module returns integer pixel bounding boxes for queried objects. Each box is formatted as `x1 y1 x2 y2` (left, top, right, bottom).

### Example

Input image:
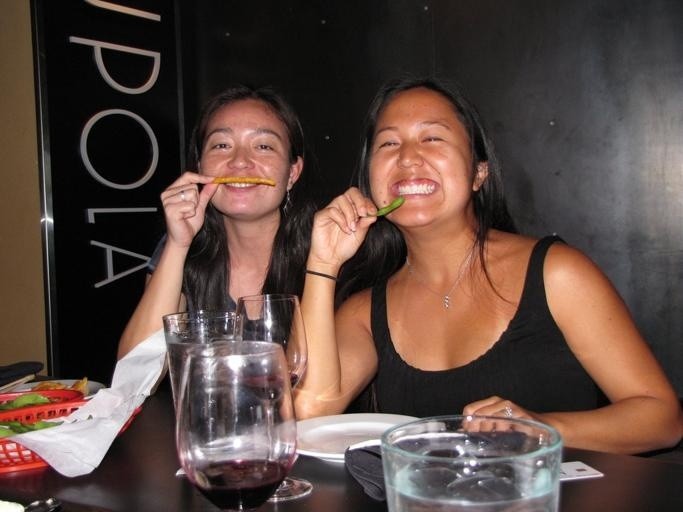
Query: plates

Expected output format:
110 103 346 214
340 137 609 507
287 412 427 468
11 379 104 399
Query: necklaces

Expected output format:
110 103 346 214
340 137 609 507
406 249 474 312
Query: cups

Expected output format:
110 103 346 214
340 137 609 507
380 415 560 511
160 313 246 416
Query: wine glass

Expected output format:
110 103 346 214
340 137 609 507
177 345 297 506
232 293 312 503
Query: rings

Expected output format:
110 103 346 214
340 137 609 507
500 405 514 421
180 191 186 202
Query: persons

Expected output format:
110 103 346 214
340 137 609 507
112 82 366 415
282 71 681 458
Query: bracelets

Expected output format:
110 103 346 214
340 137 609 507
305 269 340 282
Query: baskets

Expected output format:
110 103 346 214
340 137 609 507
0 389 83 424
0 401 141 473
